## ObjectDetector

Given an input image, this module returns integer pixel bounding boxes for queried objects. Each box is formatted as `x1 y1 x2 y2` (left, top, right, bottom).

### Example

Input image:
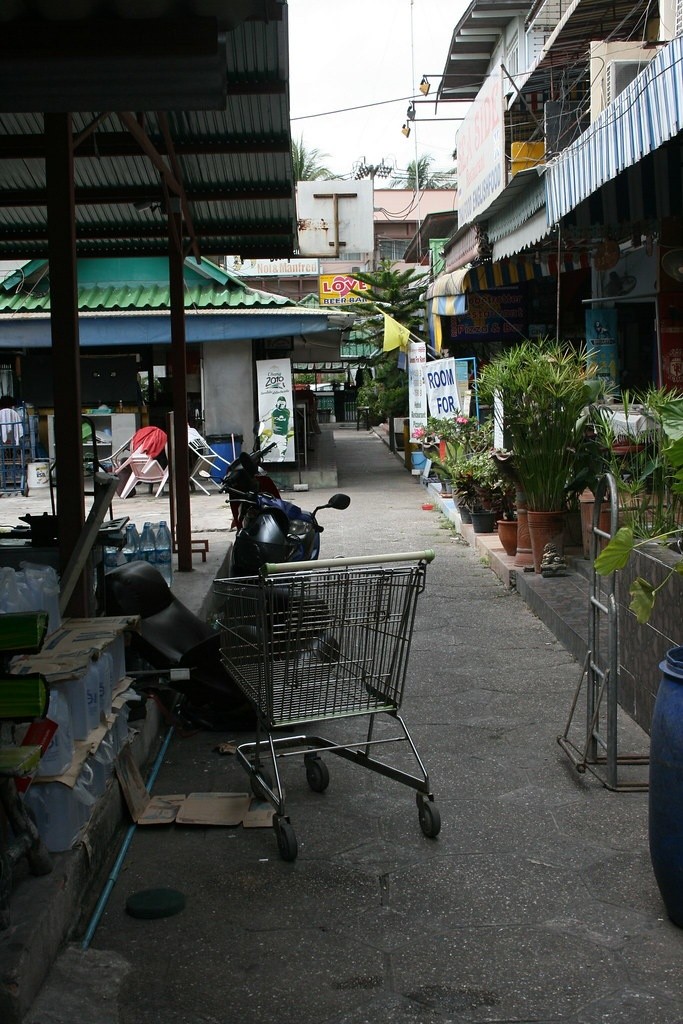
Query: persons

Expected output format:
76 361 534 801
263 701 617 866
259 396 294 462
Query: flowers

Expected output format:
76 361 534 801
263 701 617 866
412 408 496 459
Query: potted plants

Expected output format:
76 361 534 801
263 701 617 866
437 333 625 577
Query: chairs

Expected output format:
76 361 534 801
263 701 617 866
188 426 232 496
114 435 169 500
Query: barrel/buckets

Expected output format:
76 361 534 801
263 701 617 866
411 451 426 469
649 646 683 928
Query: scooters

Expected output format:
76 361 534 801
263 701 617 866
197 419 281 530
223 483 351 586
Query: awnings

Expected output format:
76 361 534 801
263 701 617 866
427 39 682 319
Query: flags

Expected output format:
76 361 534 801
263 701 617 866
383 316 410 353
423 358 462 424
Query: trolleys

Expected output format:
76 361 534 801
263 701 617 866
0 400 28 497
213 548 440 862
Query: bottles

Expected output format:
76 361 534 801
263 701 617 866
100 520 171 588
1 566 61 638
57 633 127 743
119 399 123 413
24 718 125 854
3 687 76 780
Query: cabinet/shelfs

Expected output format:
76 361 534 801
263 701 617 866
48 412 140 484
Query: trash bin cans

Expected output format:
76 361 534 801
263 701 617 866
206 431 244 485
